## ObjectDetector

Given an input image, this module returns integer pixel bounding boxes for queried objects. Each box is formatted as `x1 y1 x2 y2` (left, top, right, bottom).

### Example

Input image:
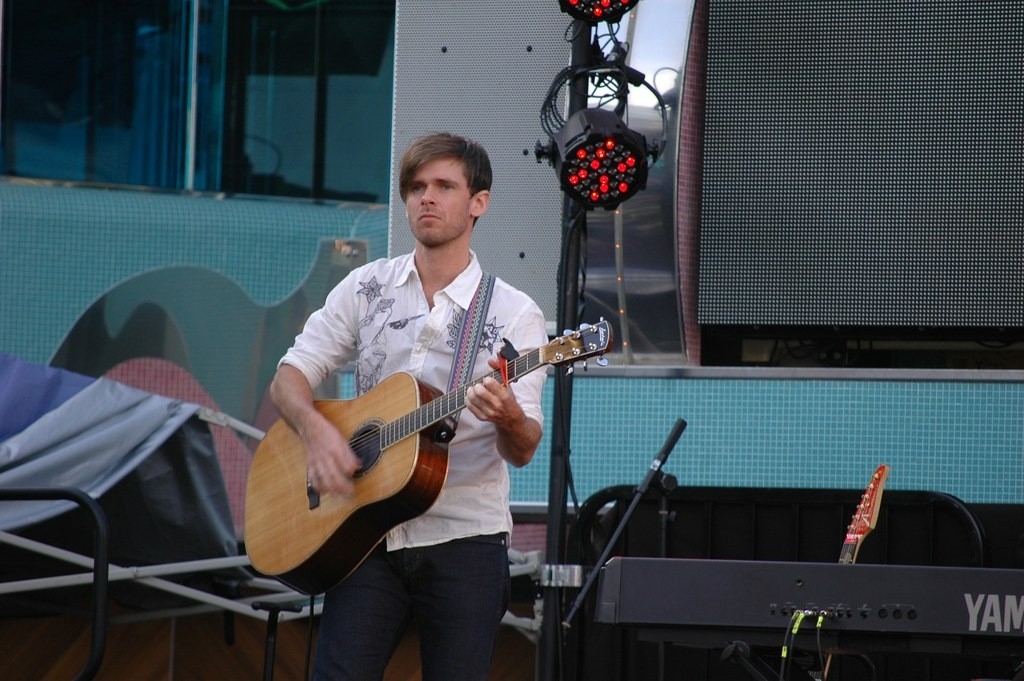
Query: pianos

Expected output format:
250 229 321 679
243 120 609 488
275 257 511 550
594 555 1023 681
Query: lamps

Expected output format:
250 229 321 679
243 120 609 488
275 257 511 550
558 0 640 28
534 107 661 211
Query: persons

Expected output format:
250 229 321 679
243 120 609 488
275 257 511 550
270 133 548 681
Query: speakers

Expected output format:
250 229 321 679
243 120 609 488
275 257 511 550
688 0 1024 372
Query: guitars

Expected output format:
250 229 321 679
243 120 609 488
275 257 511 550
837 462 890 562
243 314 614 599
